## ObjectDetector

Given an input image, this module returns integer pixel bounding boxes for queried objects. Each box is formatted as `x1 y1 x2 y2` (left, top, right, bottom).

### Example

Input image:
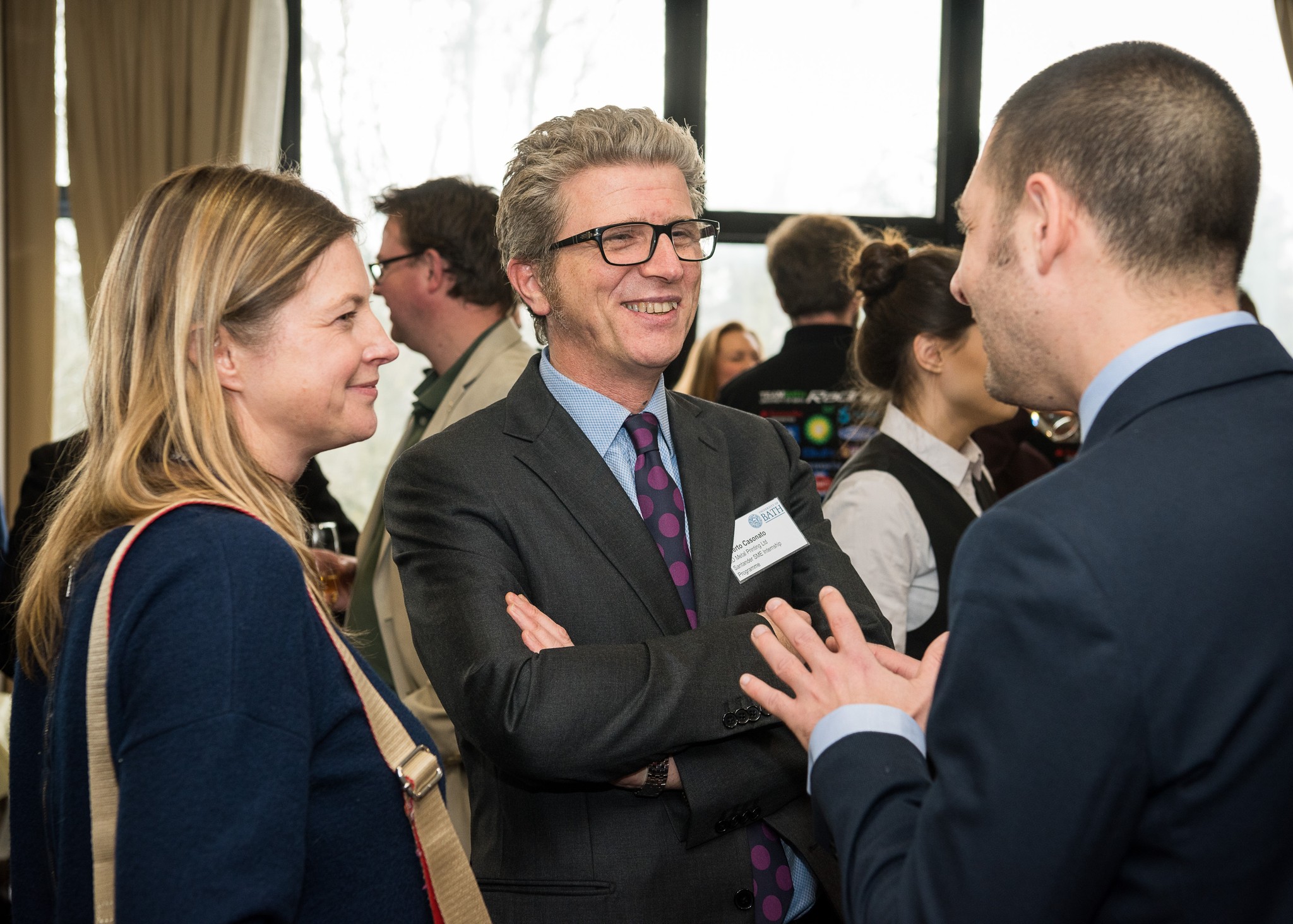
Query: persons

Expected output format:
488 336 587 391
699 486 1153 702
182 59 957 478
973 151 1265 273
11 158 448 924
970 405 1080 501
387 106 895 923
822 239 1020 660
689 321 761 403
717 215 891 501
315 176 540 860
739 41 1293 924
0 428 360 677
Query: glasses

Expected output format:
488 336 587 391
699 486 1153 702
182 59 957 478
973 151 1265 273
368 248 424 285
552 216 720 265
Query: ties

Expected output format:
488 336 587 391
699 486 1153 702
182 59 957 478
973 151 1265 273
624 411 795 924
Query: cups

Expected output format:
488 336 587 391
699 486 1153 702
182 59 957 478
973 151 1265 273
1025 408 1079 442
306 522 340 606
